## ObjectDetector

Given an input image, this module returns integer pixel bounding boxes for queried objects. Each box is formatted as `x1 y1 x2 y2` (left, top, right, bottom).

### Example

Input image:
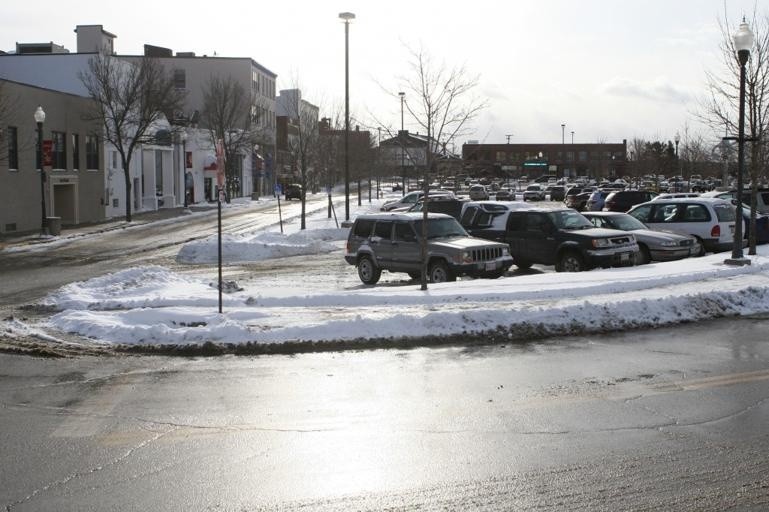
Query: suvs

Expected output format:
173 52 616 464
343 210 515 285
285 182 303 202
457 199 640 274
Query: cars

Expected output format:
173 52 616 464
576 208 702 267
717 194 768 244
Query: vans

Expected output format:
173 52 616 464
623 198 749 254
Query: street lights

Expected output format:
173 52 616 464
674 131 682 185
723 15 754 267
32 103 52 237
336 10 357 228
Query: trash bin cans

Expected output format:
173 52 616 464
46 217 62 235
252 192 259 200
